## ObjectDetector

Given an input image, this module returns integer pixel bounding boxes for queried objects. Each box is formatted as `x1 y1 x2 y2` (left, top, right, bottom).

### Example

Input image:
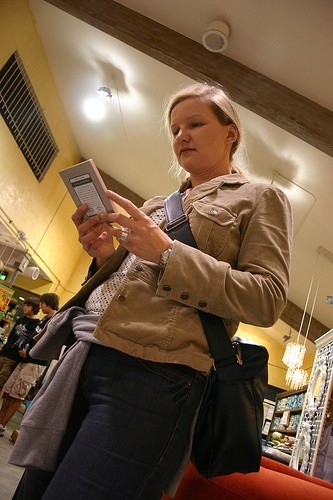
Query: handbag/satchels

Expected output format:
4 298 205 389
190 340 269 478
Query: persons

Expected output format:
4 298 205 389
10 84 293 500
0 293 59 437
0 298 40 390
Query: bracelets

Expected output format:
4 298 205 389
160 245 173 270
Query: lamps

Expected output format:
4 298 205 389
280 246 333 391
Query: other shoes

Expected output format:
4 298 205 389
0 424 6 437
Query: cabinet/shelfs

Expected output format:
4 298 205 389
266 383 309 455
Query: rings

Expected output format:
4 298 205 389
120 229 129 240
